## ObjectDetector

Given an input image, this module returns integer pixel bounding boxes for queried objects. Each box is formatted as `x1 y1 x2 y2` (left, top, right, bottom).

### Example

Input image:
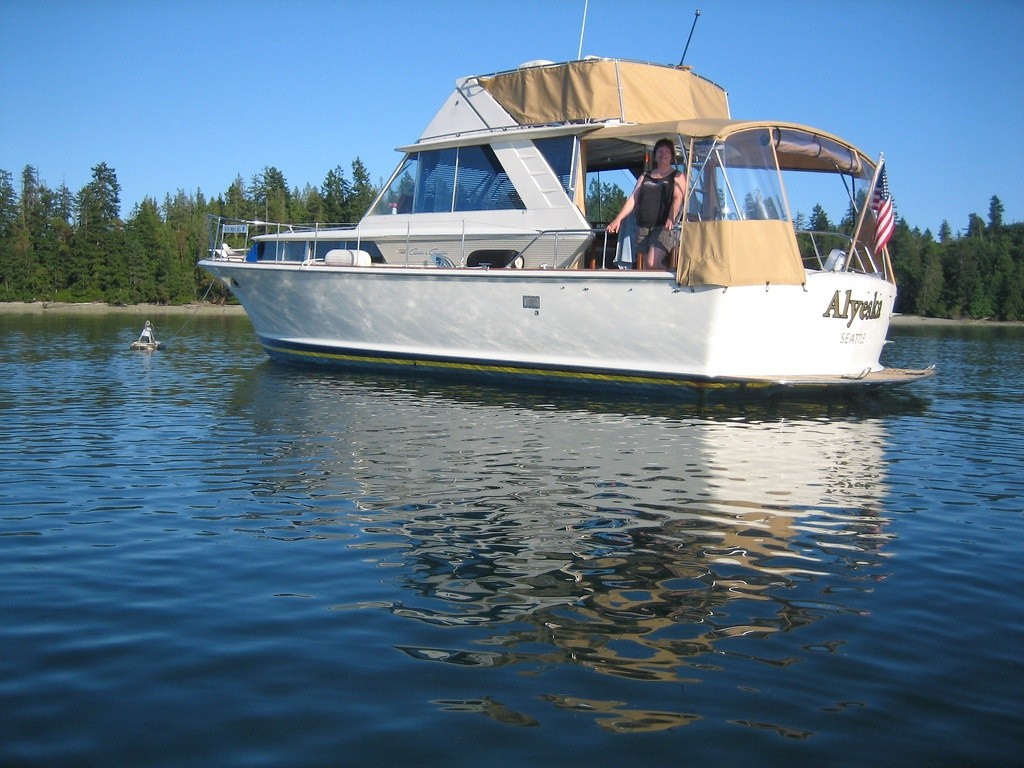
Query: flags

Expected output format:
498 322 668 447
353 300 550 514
868 164 896 255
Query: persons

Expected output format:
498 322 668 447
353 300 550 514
606 138 686 270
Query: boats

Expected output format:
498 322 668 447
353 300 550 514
129 321 166 350
196 0 937 399
